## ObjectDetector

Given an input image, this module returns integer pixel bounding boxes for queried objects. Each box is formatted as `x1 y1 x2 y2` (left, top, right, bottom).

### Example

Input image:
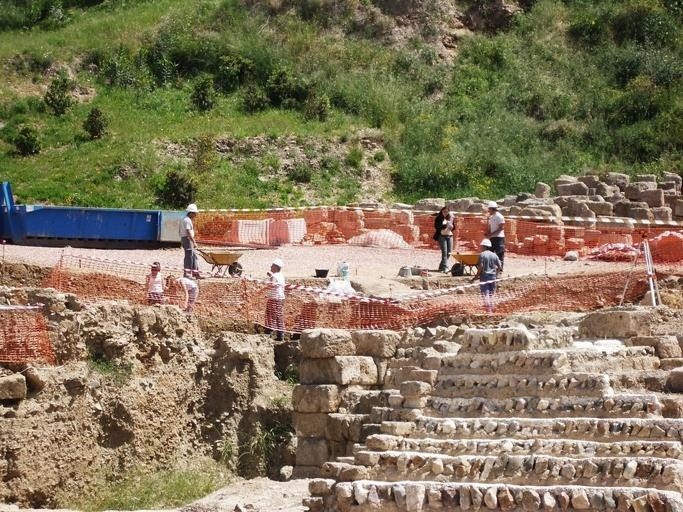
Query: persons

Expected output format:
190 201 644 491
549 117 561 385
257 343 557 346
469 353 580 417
485 200 507 273
256 259 287 341
166 274 199 314
179 202 206 281
432 206 456 275
473 238 502 312
145 261 172 306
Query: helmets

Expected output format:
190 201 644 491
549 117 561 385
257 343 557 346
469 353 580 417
486 201 499 208
186 204 199 214
272 258 284 268
479 238 492 247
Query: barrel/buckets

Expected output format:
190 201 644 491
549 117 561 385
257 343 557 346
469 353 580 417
336 261 350 276
315 268 330 278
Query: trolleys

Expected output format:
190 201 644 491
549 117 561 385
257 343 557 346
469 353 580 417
191 241 243 278
447 249 482 277
